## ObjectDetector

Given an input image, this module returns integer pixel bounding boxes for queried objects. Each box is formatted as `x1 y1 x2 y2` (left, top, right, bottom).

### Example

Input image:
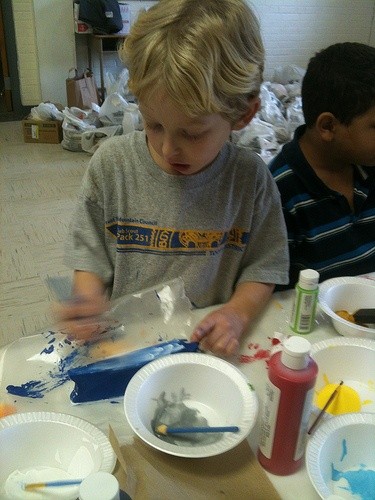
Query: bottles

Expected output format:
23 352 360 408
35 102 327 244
76 471 132 500
257 336 319 475
290 268 320 335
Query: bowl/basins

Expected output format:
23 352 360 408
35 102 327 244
307 336 375 420
124 353 259 459
0 410 117 500
317 276 375 340
304 412 375 500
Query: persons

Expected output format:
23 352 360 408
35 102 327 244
268 42 375 288
58 0 290 358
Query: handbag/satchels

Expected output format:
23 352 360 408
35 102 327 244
78 0 124 33
103 69 138 103
29 92 146 156
230 64 308 165
73 2 93 34
66 67 101 109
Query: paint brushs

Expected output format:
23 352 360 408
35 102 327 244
307 380 343 436
157 425 239 433
26 480 83 487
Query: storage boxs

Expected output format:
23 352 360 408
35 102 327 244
22 114 63 144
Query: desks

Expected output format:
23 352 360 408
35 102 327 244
0 275 375 500
84 33 127 105
